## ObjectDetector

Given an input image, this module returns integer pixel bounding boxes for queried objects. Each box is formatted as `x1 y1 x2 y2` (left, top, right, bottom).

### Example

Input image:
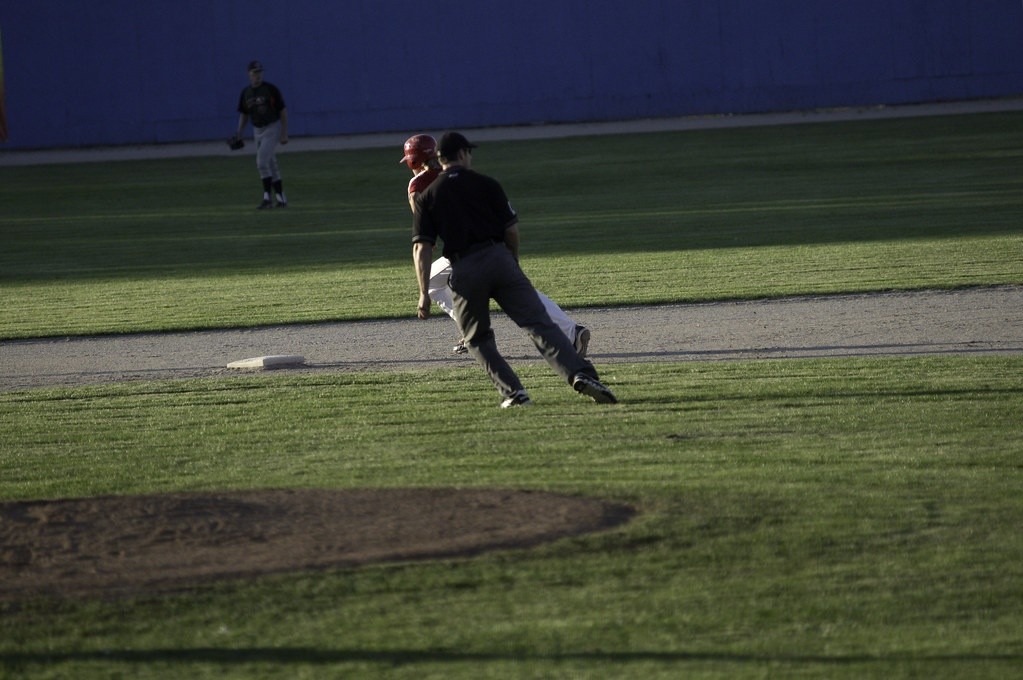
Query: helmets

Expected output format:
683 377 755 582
399 134 437 169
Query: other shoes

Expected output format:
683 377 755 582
256 199 273 210
273 201 286 210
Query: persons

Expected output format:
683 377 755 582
235 61 288 210
411 132 617 409
399 134 590 360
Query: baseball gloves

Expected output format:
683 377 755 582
225 132 245 153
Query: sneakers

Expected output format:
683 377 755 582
573 324 590 360
572 372 617 404
453 329 496 354
501 388 531 408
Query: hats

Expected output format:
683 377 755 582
246 60 266 72
434 132 479 157
